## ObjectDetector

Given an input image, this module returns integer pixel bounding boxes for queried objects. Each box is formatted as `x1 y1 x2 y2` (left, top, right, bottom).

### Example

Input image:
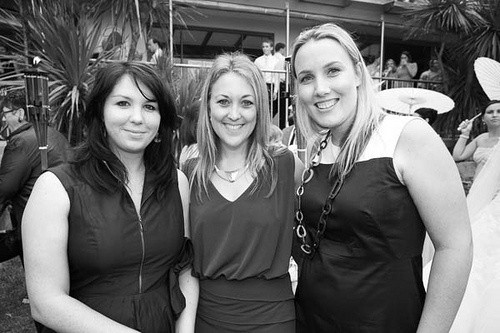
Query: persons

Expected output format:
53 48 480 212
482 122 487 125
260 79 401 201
21 61 199 333
294 24 474 333
0 87 74 269
148 37 500 180
179 52 306 333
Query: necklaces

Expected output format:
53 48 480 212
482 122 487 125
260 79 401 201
297 128 355 253
212 161 252 182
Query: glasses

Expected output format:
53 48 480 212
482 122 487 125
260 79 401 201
0 107 19 118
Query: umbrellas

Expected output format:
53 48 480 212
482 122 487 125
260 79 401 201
376 88 454 115
473 56 500 100
